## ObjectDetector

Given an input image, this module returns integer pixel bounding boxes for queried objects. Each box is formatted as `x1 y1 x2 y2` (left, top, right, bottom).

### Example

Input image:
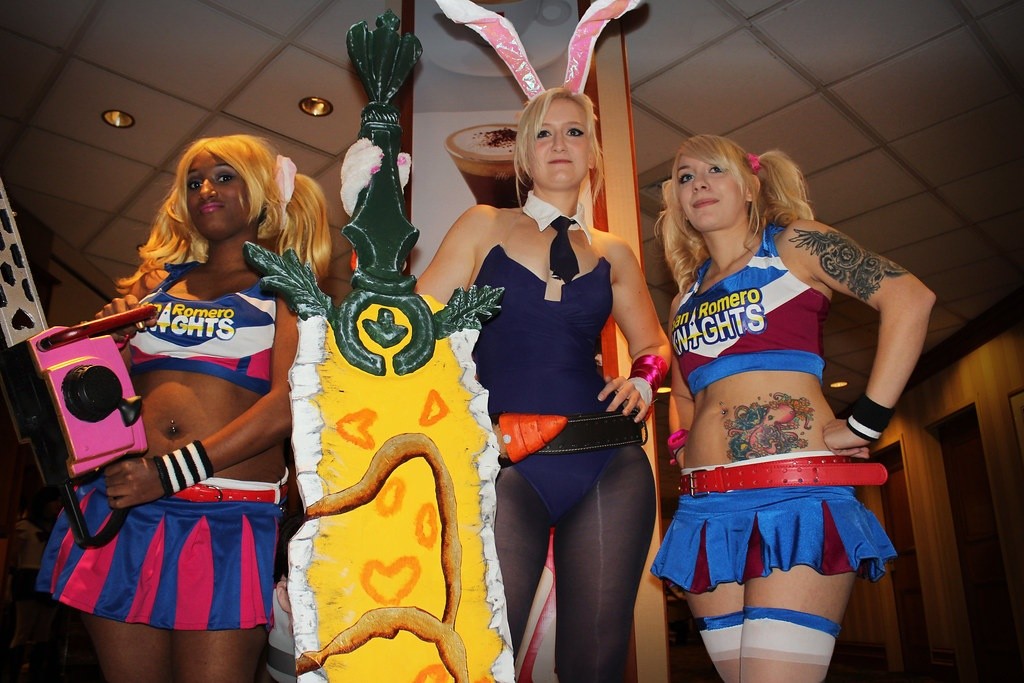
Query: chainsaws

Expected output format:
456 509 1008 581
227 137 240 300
0 177 158 549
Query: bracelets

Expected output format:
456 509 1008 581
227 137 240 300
846 394 894 441
156 440 214 499
628 376 653 407
666 428 690 466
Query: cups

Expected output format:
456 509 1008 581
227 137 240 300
446 123 533 208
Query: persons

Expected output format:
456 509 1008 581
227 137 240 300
32 135 333 683
650 132 941 682
411 87 672 683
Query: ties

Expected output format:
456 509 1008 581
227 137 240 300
549 216 580 284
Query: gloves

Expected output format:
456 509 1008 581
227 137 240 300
340 138 412 272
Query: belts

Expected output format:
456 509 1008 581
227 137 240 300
491 411 643 464
679 454 888 498
173 483 288 503
